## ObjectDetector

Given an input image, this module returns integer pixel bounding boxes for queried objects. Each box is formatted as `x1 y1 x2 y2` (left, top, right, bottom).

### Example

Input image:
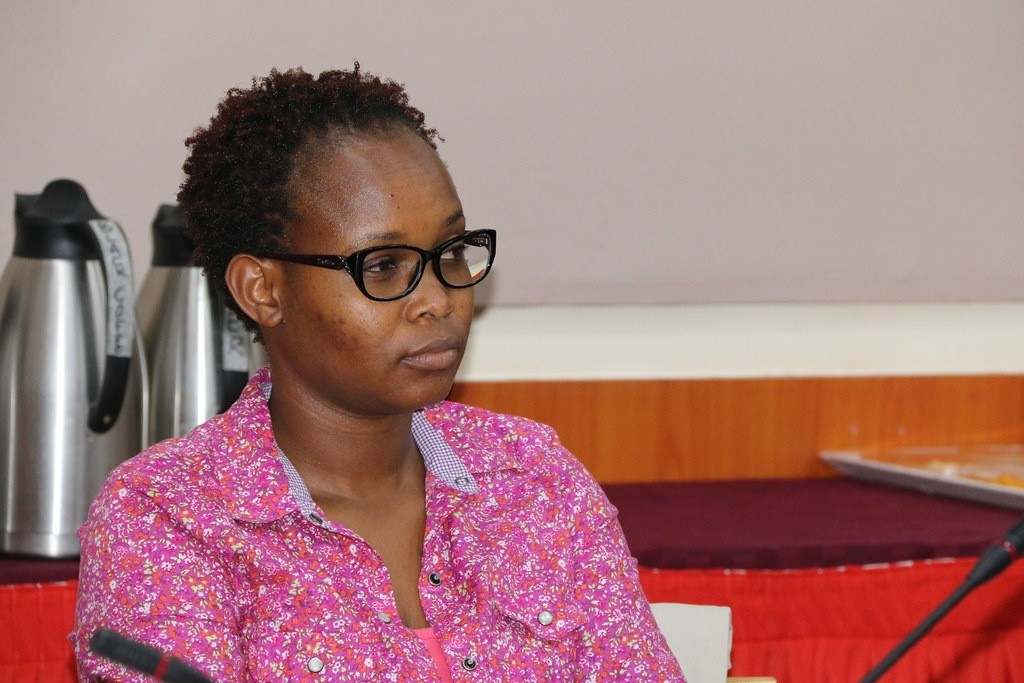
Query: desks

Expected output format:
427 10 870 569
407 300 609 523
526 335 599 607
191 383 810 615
0 474 1024 683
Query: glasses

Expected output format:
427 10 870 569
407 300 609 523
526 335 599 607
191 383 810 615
253 228 496 301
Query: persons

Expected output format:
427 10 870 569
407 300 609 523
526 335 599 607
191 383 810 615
75 63 686 683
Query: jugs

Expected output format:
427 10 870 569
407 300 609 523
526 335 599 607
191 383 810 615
129 204 266 454
0 178 145 560
